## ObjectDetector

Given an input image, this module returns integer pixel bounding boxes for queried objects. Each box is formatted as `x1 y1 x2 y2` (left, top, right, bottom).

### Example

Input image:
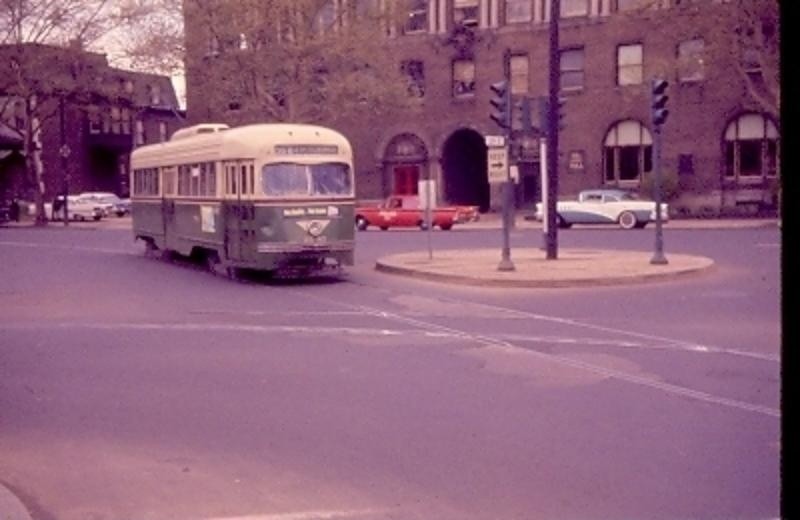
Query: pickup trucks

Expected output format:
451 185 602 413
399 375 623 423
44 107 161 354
355 195 481 231
534 188 671 231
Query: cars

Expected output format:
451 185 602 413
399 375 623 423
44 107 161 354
43 191 132 222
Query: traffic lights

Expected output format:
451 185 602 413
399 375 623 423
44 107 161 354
555 98 568 134
488 79 513 129
649 79 670 131
514 95 531 132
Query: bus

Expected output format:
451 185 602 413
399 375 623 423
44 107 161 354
129 122 356 284
